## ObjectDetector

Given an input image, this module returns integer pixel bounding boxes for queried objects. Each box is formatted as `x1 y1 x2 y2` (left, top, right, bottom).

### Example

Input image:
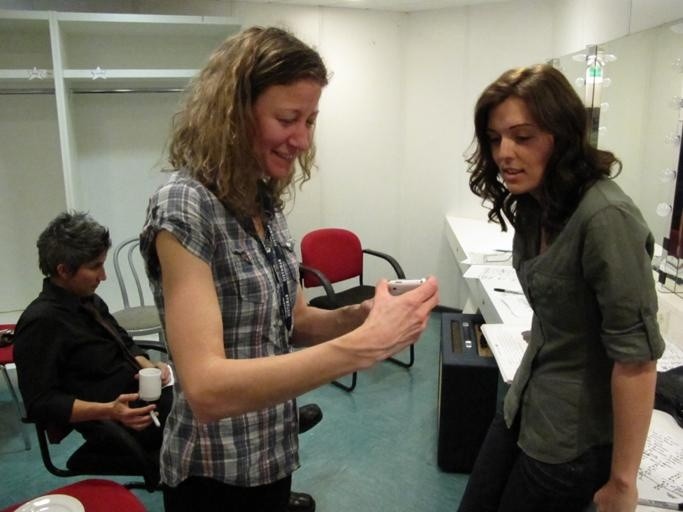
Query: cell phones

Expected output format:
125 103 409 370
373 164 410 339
387 280 425 296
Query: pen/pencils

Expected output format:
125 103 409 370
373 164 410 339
494 288 523 293
638 498 683 511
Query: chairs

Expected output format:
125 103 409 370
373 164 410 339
0 479 147 512
110 237 167 353
32 425 161 491
297 228 414 392
1 323 32 452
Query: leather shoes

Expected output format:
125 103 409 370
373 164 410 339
289 492 315 512
299 403 323 432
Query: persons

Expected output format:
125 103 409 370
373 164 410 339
140 27 439 512
456 65 666 512
14 210 323 512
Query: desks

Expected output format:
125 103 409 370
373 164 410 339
442 215 682 511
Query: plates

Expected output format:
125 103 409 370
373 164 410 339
13 494 87 512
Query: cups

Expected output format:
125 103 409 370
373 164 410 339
140 368 162 402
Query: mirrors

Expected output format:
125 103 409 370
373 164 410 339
549 17 683 253
